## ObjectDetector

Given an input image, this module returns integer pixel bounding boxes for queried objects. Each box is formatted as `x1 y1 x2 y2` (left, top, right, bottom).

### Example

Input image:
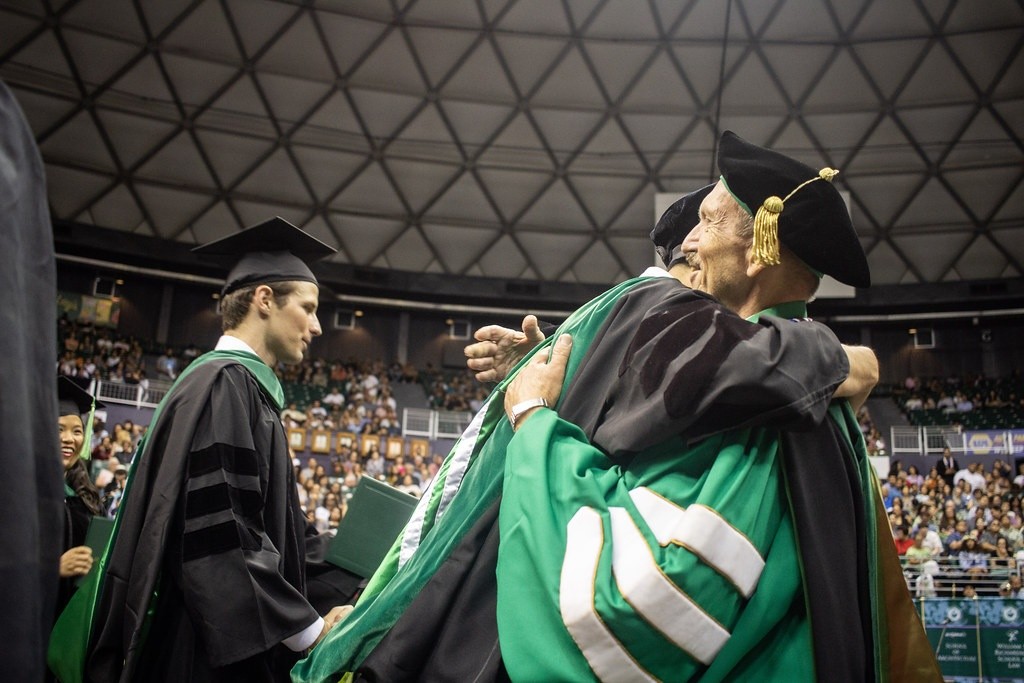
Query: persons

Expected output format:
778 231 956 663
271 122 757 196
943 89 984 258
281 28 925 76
49 306 1024 611
469 133 885 683
346 179 884 683
91 244 356 683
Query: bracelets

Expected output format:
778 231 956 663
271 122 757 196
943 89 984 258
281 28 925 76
509 397 552 432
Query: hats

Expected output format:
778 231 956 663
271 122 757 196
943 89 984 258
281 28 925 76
58 375 107 419
190 214 338 300
650 179 719 272
716 128 872 288
